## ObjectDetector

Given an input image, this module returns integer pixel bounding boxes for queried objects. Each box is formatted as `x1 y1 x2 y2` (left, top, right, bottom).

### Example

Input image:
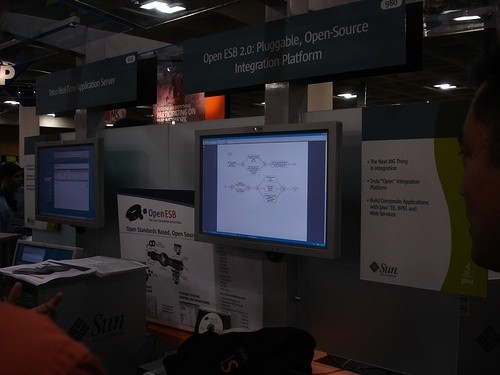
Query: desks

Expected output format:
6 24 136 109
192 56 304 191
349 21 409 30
0 254 154 375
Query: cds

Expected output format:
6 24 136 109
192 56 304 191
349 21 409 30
199 313 223 334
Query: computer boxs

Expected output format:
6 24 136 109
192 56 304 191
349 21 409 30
0 267 147 375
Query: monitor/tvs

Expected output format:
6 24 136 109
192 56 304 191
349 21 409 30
12 239 83 265
35 137 104 229
194 120 343 260
